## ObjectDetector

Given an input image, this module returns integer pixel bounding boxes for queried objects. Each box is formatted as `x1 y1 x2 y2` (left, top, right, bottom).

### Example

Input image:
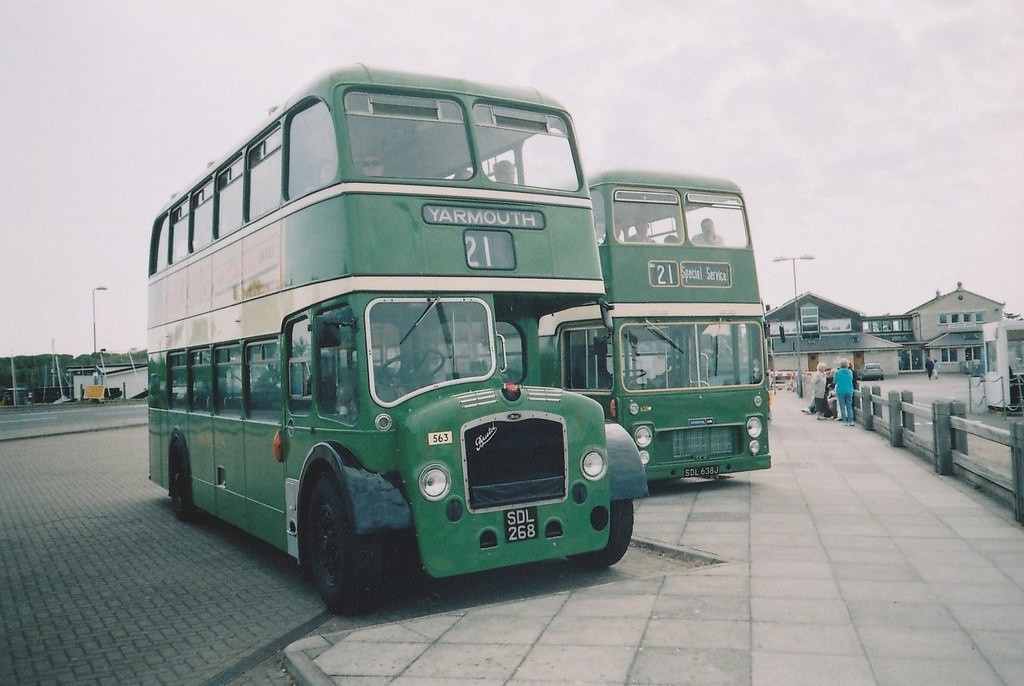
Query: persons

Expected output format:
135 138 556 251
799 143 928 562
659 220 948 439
925 357 940 381
615 219 623 242
801 358 857 426
362 151 385 178
319 159 337 183
664 235 677 243
625 218 656 243
691 219 724 246
453 171 473 179
493 160 516 184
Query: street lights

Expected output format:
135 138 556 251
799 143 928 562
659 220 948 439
771 254 816 400
92 286 108 384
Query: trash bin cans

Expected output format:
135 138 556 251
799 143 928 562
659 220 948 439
960 361 974 373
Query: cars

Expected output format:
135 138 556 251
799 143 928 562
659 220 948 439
856 363 884 381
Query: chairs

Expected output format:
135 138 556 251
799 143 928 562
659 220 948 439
193 394 243 418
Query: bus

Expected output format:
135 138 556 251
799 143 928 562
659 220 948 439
448 169 772 478
147 65 650 613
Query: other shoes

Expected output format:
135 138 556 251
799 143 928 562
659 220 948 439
801 409 816 415
817 417 824 421
825 415 834 420
841 421 855 426
834 418 843 421
